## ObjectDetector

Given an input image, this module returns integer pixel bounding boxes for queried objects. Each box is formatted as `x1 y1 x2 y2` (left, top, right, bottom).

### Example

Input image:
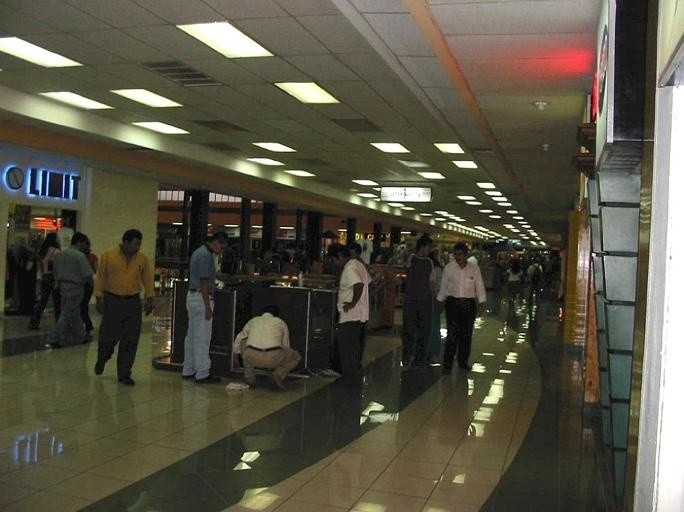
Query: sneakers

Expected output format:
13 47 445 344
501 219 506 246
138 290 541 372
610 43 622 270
267 375 284 390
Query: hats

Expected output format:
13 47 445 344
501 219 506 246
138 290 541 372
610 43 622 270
325 243 345 256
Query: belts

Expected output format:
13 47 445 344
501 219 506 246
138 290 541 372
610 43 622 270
188 288 212 295
105 291 139 300
58 280 76 285
247 345 281 351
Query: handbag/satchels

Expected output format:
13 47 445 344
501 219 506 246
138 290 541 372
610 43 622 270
48 248 57 271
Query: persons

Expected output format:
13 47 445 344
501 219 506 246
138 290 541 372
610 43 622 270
261 240 324 274
232 304 302 390
326 241 374 385
181 231 230 384
95 229 155 386
400 233 487 376
7 231 98 348
506 255 554 301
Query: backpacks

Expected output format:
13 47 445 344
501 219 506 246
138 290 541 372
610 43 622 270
531 268 542 285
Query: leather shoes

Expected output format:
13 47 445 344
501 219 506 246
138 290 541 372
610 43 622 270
441 365 453 375
119 376 134 386
183 373 220 383
246 383 258 390
458 364 472 371
95 358 105 374
